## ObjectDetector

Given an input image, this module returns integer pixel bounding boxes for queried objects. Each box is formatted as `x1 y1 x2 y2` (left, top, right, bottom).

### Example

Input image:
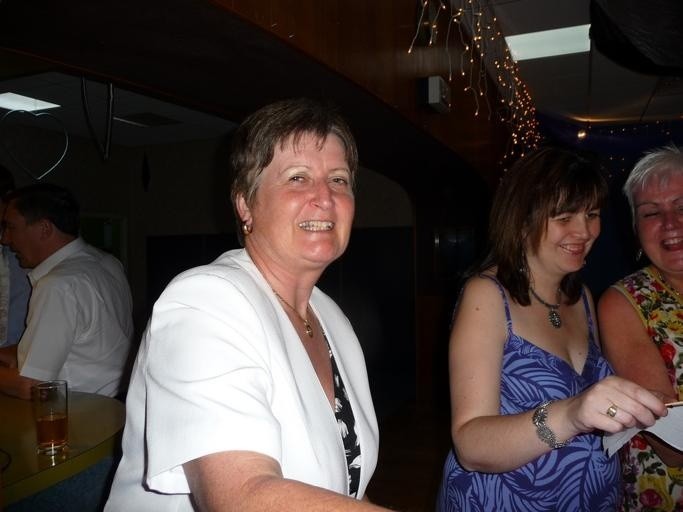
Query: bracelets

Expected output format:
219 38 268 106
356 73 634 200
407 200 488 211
532 400 574 448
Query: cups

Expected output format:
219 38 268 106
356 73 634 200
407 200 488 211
31 379 69 456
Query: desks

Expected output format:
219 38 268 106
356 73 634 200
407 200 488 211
0 383 126 511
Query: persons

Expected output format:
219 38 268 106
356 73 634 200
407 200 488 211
100 99 400 512
595 147 683 511
438 145 671 510
0 182 133 512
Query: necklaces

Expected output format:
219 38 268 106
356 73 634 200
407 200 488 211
657 272 682 300
527 285 565 329
270 286 315 339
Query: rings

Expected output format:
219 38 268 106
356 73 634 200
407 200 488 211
606 404 620 418
